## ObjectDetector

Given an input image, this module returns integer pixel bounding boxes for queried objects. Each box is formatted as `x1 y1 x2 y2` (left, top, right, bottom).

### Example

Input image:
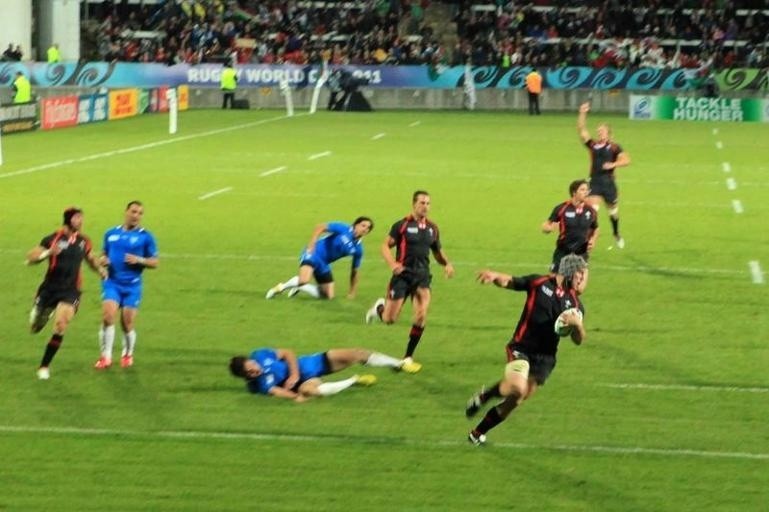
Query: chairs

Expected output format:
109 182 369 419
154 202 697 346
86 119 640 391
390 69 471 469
350 298 423 386
613 232 625 249
36 352 134 380
465 392 488 448
265 282 301 300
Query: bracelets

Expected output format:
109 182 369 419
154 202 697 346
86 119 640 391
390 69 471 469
137 256 145 264
39 250 50 260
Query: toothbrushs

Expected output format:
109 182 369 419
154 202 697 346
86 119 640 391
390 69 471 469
555 310 583 338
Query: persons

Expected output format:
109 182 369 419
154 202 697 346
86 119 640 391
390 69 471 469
697 68 720 97
333 72 369 110
324 68 346 110
47 42 62 63
229 346 422 402
265 216 374 301
2 51 12 62
576 100 630 250
523 68 544 113
365 191 454 374
220 64 238 110
94 200 160 368
541 179 599 274
93 1 766 69
25 206 109 380
463 253 589 447
3 44 13 56
10 72 32 105
11 44 22 61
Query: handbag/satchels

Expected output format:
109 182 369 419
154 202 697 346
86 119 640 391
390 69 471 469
39 250 50 260
137 256 145 264
